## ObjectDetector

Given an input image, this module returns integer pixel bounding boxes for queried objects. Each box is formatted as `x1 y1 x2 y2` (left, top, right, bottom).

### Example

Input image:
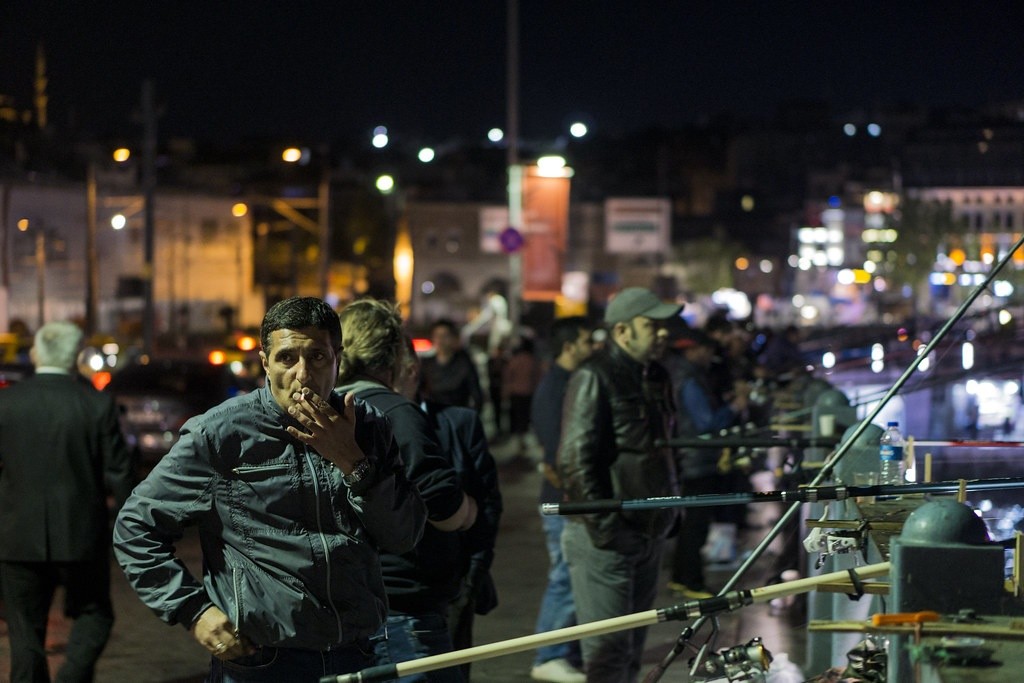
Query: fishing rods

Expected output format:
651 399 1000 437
319 536 1017 683
641 234 1024 683
540 475 1024 516
650 299 1024 453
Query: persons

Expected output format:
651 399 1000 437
487 286 802 683
113 296 503 682
1 320 133 682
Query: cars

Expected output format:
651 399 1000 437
1 325 261 465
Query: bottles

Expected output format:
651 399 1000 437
879 422 908 502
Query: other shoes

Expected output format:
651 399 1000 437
531 657 589 683
666 584 719 601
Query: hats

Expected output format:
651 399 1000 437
603 286 685 322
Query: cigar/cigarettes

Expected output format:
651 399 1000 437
301 393 305 400
